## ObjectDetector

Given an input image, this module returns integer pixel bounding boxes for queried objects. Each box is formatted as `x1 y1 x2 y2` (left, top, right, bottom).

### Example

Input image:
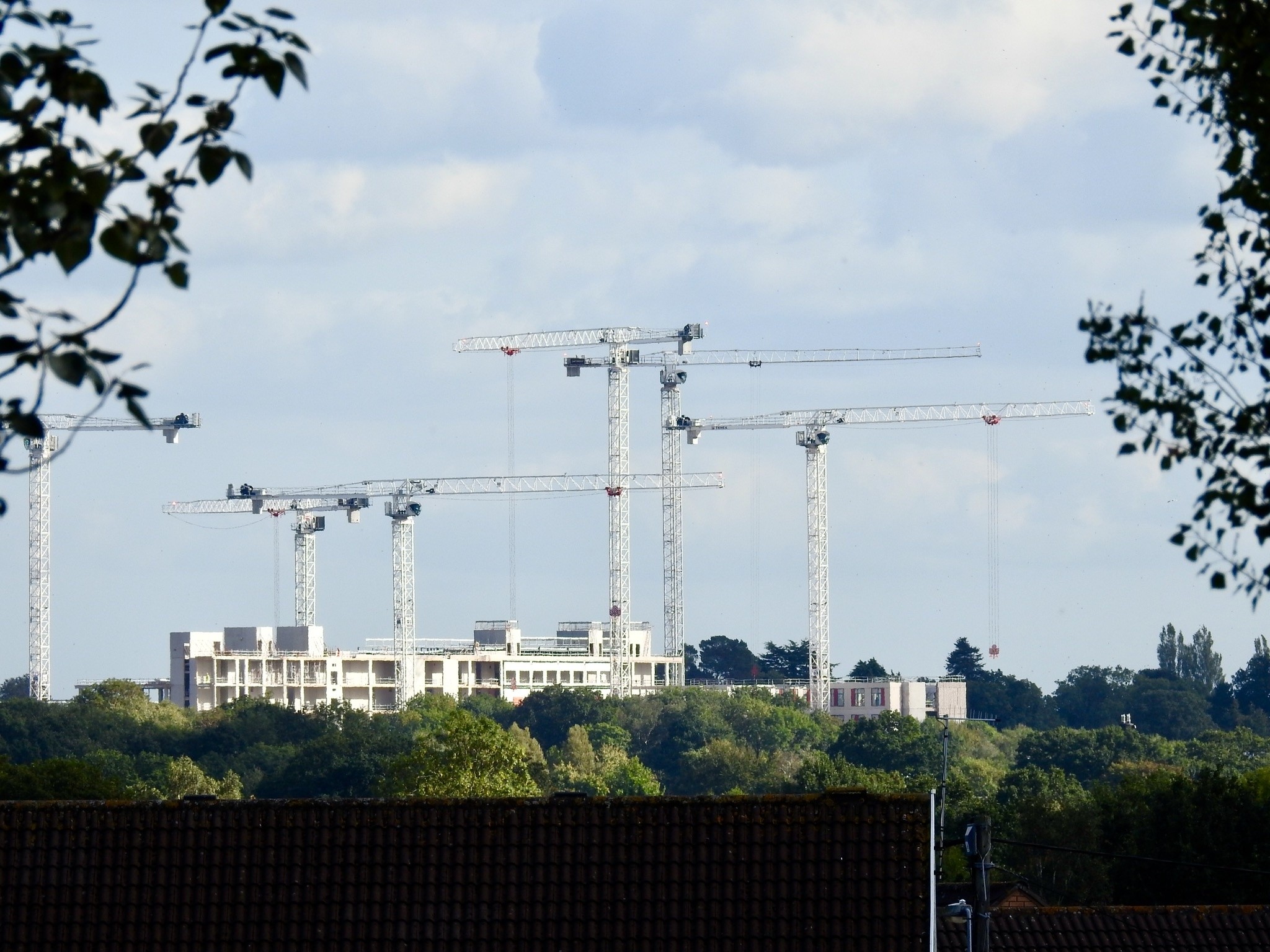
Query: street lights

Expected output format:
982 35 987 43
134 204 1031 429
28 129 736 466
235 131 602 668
944 899 972 952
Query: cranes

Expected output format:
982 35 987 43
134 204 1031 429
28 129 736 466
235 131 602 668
1 413 199 707
161 327 1097 716
